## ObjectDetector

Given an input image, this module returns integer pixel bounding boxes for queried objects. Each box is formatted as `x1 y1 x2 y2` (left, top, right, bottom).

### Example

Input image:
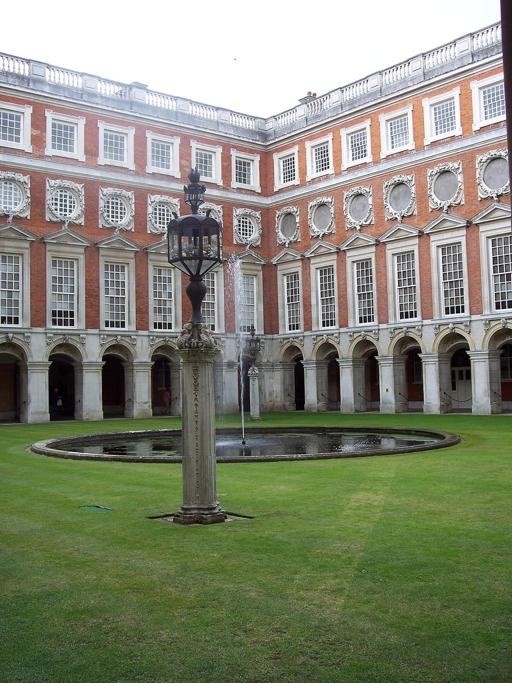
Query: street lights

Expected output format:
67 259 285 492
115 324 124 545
247 323 262 419
163 167 235 524
